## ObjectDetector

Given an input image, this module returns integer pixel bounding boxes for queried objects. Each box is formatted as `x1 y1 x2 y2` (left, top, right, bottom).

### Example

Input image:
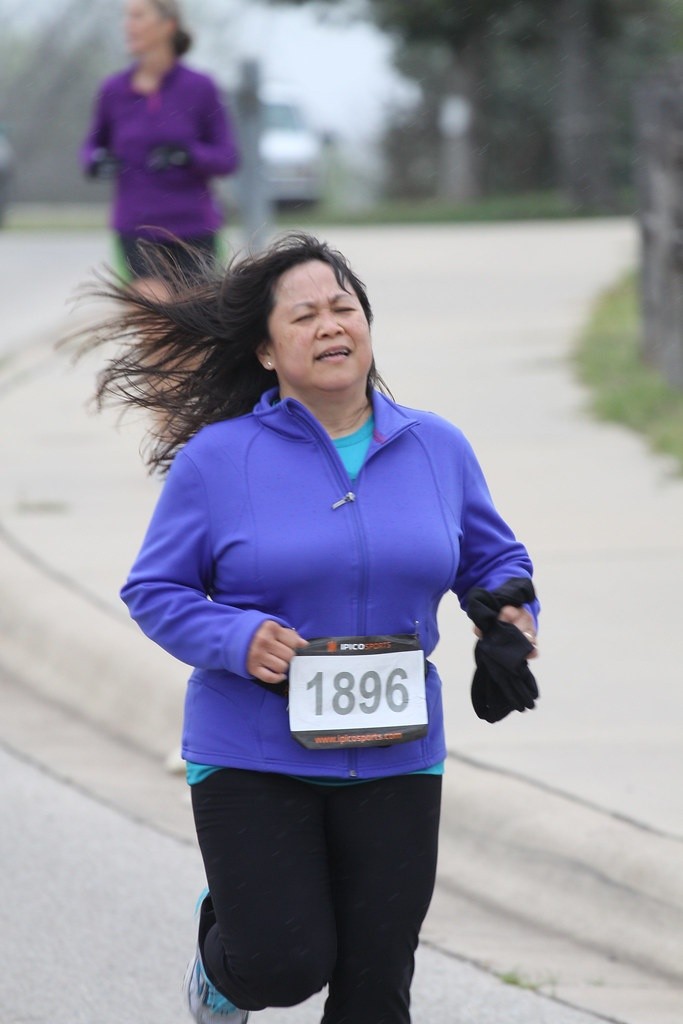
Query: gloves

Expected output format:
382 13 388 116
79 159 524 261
160 145 194 169
87 152 116 182
467 588 539 724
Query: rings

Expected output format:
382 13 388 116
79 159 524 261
524 632 534 639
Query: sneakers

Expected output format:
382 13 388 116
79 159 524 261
187 887 249 1024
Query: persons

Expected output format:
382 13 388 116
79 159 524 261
80 0 238 436
81 242 540 1024
